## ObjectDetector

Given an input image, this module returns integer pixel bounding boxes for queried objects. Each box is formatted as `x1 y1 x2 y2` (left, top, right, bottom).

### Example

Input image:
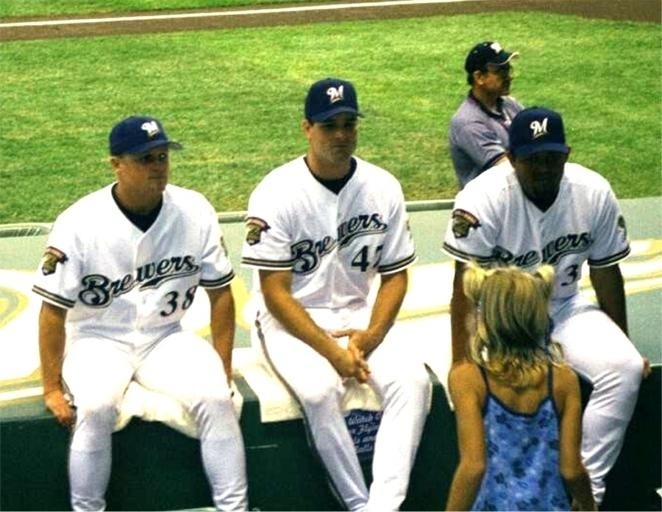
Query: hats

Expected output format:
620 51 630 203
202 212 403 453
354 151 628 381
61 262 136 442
304 77 365 122
508 105 569 161
465 40 520 71
108 117 182 156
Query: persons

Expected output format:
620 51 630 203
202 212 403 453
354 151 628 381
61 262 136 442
241 76 433 510
31 115 251 510
444 259 599 510
445 106 652 509
448 42 525 189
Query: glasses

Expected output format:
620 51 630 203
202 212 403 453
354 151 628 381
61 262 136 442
484 65 514 73
